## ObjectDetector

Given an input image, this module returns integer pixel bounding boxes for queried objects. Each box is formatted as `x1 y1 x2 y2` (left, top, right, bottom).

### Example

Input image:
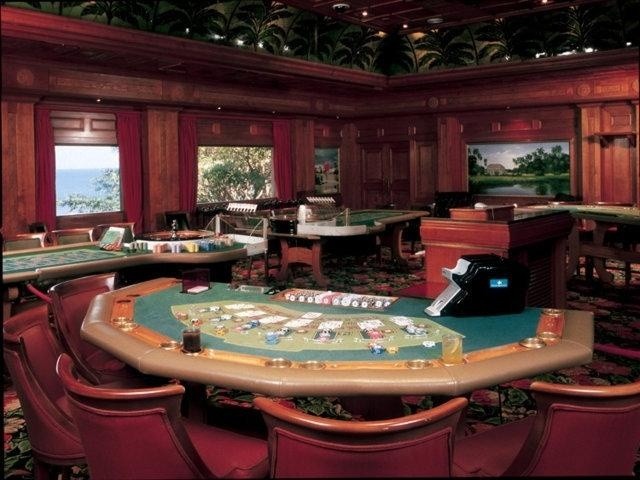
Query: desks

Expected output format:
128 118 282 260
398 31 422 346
235 208 431 290
3 234 249 306
80 275 595 443
419 206 573 298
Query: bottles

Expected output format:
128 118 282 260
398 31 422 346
535 307 565 347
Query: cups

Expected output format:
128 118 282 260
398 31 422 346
182 323 202 353
442 333 463 365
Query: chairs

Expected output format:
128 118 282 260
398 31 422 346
548 200 639 311
47 272 175 388
2 211 135 305
453 342 639 478
161 197 298 275
253 395 468 478
55 354 271 479
0 303 137 478
370 203 438 259
